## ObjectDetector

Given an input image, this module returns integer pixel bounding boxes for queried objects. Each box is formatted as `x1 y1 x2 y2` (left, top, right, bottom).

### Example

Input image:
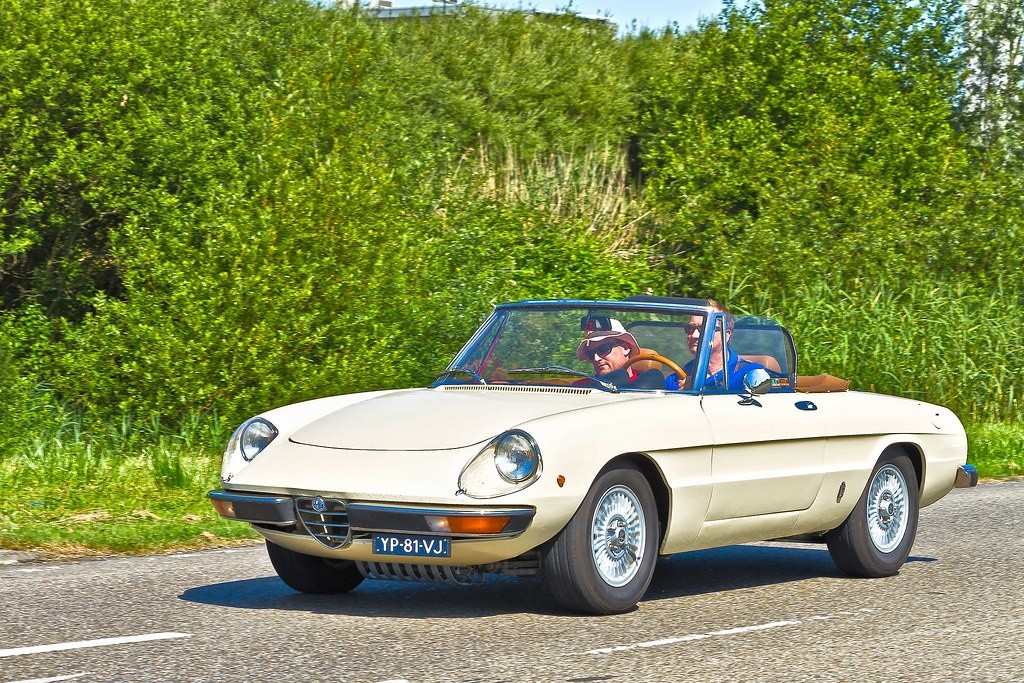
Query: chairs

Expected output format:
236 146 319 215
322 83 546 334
740 355 782 372
630 348 662 372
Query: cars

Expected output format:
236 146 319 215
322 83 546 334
207 294 980 615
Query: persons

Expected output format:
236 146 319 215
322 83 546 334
571 318 666 392
664 299 769 394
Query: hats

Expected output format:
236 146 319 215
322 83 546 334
577 318 641 361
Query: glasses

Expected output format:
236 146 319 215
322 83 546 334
683 323 722 335
585 341 624 361
